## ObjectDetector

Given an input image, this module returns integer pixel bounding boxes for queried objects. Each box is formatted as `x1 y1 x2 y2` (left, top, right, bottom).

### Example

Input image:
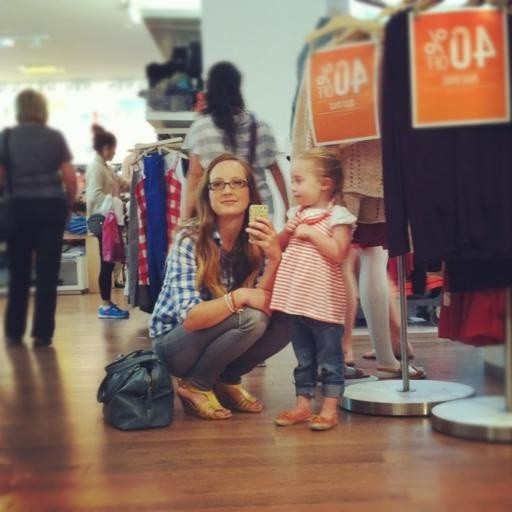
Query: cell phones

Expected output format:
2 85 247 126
248 204 269 242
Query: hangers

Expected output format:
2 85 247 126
326 7 417 50
305 11 388 46
411 1 443 15
373 2 411 31
131 145 188 165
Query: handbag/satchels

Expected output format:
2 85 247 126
96 348 175 431
99 182 126 264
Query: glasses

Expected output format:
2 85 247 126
207 177 249 192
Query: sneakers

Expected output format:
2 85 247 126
308 413 341 431
96 305 130 319
274 408 313 427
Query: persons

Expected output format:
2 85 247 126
344 244 415 364
182 61 289 227
342 222 428 378
85 123 131 320
147 151 290 422
274 147 357 431
0 88 77 347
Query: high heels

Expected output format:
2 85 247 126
174 378 232 422
216 380 262 414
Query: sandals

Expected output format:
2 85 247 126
339 363 371 380
375 363 428 382
